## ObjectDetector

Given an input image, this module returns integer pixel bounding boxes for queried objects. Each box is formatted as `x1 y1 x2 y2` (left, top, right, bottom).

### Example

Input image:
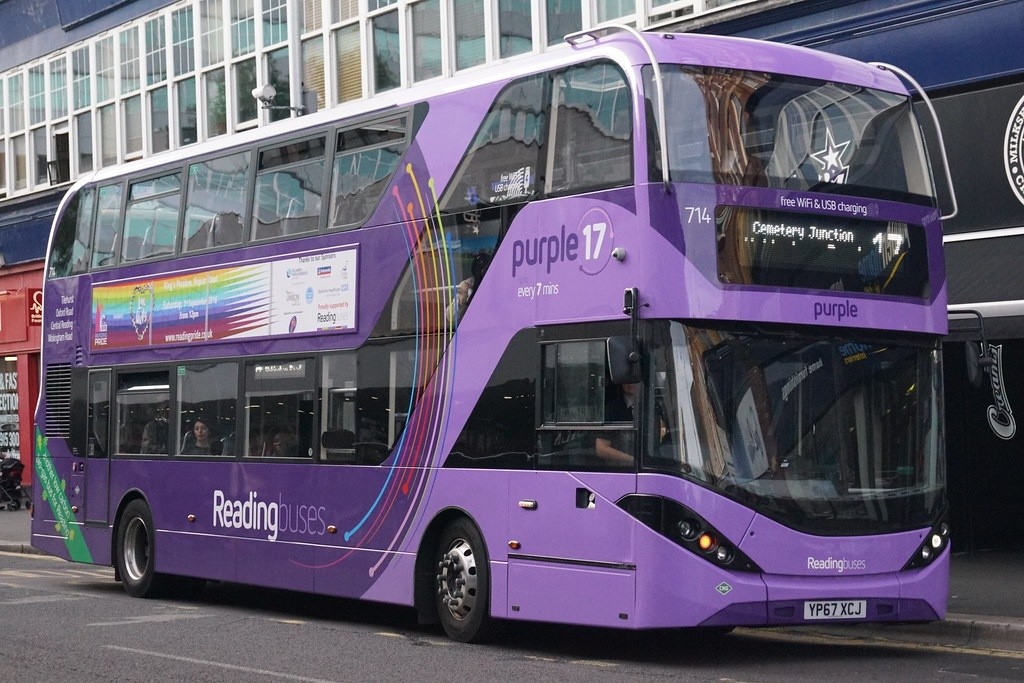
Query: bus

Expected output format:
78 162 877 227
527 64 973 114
30 25 994 649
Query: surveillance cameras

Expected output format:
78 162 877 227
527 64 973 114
250 83 277 102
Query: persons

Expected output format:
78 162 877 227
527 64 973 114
140 395 295 457
595 367 669 467
446 253 493 327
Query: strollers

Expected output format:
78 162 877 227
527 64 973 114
0 458 32 511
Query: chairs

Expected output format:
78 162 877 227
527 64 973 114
219 433 236 454
107 194 309 270
182 431 195 454
